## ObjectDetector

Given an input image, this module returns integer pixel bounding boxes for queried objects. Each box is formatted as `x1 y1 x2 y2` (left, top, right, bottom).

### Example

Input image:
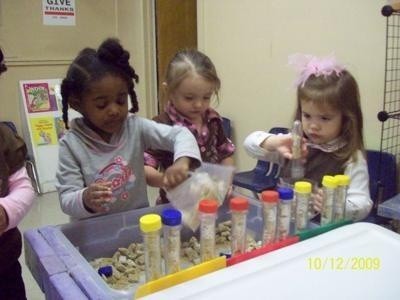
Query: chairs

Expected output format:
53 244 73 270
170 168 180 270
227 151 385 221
363 150 396 225
233 127 291 200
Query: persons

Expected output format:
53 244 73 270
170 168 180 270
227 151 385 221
241 52 376 224
56 36 203 224
0 48 39 300
143 46 238 204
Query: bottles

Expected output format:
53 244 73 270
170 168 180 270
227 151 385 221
289 120 306 180
137 172 355 291
